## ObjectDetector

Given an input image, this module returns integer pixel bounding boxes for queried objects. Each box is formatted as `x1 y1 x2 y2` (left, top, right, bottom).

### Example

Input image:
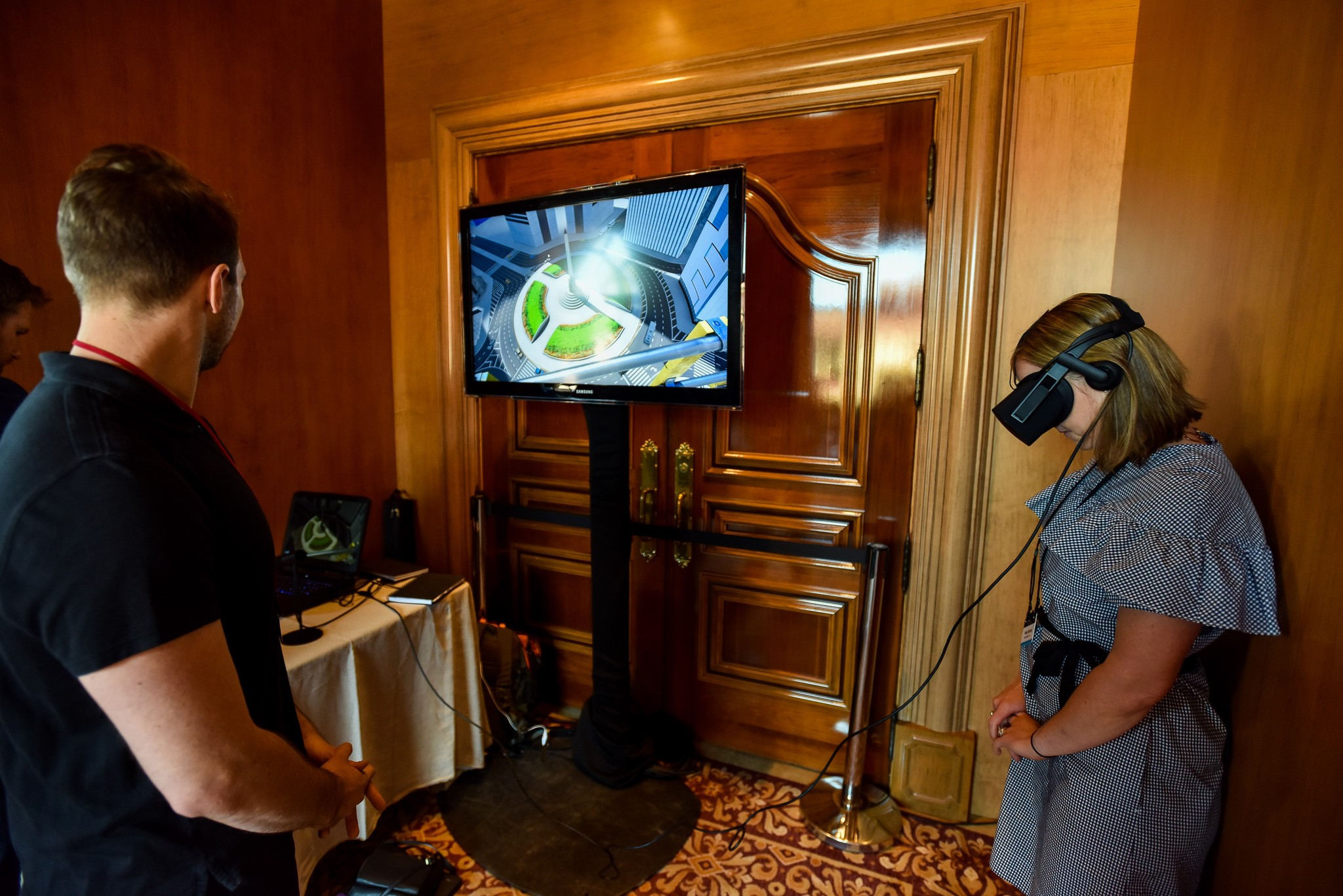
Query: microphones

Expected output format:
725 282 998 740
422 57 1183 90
293 549 306 596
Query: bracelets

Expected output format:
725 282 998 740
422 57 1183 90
1030 729 1058 758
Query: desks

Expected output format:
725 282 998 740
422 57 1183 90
281 573 492 896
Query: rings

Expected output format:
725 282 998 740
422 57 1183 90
998 727 1007 736
990 710 995 715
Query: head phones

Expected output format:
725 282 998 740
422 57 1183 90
1057 314 1146 392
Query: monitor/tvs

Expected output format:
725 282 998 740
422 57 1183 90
459 166 750 409
284 490 370 575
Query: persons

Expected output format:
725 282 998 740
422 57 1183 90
0 140 389 896
0 259 54 440
988 293 1281 896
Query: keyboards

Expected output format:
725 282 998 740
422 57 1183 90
272 573 331 596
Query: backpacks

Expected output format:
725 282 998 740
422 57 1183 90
475 619 555 748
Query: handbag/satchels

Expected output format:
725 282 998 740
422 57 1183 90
379 489 428 574
